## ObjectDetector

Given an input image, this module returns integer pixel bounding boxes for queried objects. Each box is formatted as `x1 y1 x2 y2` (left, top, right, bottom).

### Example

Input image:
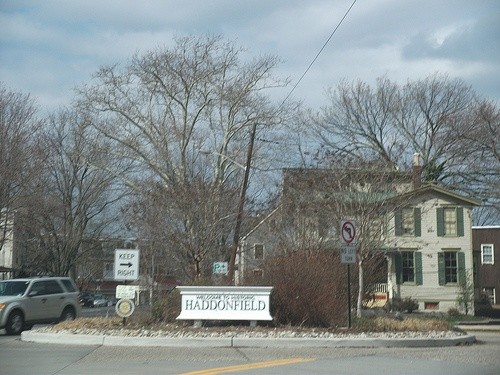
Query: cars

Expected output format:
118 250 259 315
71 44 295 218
78 291 120 308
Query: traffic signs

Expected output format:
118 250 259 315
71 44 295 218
114 248 140 281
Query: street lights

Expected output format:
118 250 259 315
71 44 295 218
199 149 251 284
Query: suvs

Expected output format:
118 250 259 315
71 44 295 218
0 276 82 337
